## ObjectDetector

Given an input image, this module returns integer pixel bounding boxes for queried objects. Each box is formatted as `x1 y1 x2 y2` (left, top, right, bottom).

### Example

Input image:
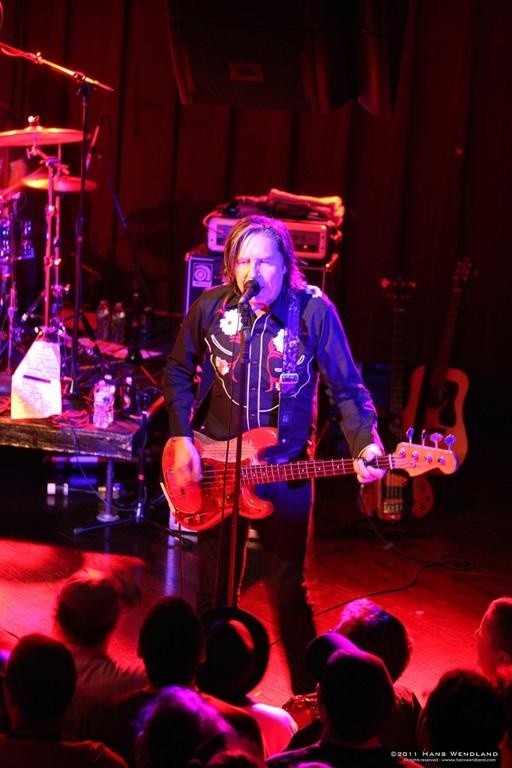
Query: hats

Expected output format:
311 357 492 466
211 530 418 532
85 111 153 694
301 631 396 721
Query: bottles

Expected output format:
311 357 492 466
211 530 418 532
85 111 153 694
95 293 153 347
94 375 113 431
122 376 149 415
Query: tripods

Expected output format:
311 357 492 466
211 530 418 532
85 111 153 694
0 42 195 550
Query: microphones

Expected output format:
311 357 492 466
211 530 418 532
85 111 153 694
238 280 260 306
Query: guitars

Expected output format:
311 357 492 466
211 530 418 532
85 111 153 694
402 258 480 476
358 279 433 523
161 426 458 531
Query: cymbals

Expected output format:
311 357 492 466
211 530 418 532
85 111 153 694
0 125 91 147
22 174 97 193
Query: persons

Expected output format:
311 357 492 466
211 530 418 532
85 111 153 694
163 214 387 707
2 583 511 767
